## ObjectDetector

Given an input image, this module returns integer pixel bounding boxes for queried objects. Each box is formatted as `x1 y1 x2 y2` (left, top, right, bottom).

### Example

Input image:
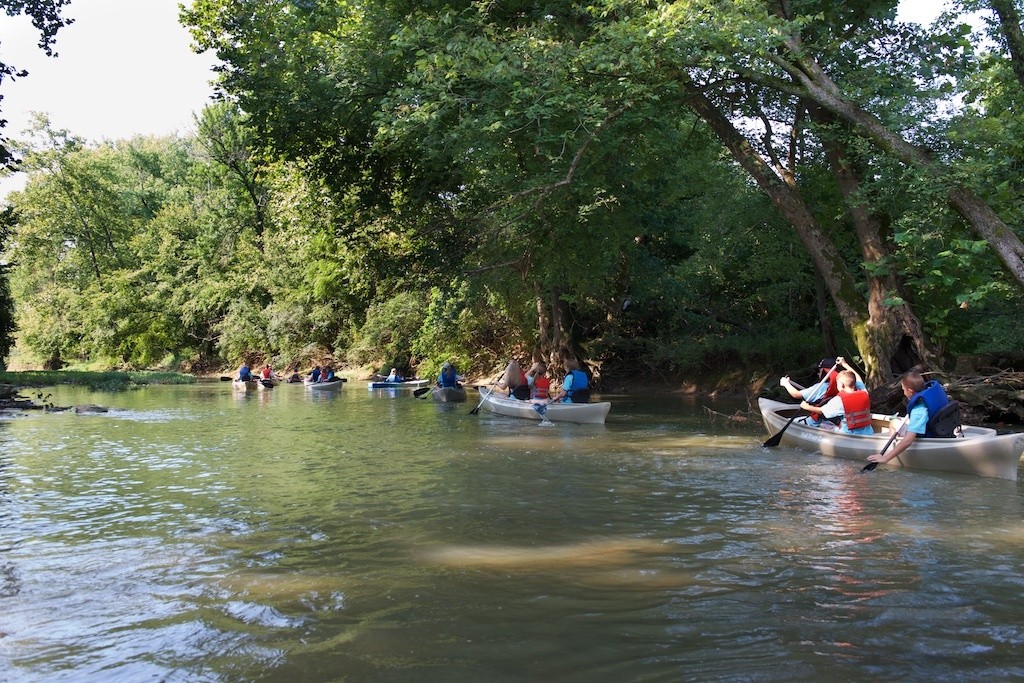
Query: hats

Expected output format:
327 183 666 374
443 362 450 370
818 358 836 368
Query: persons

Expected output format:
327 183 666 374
437 361 466 390
309 365 334 384
495 359 551 399
384 367 401 382
866 368 949 463
552 358 590 403
780 357 874 435
239 362 300 381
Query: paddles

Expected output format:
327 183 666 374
763 362 838 447
413 386 434 398
861 413 909 472
532 399 555 416
784 374 807 390
219 374 273 389
468 371 505 415
420 384 439 400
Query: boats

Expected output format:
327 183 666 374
759 398 1024 481
304 377 342 390
431 387 467 403
367 380 430 388
232 375 284 392
478 387 611 424
287 378 302 383
